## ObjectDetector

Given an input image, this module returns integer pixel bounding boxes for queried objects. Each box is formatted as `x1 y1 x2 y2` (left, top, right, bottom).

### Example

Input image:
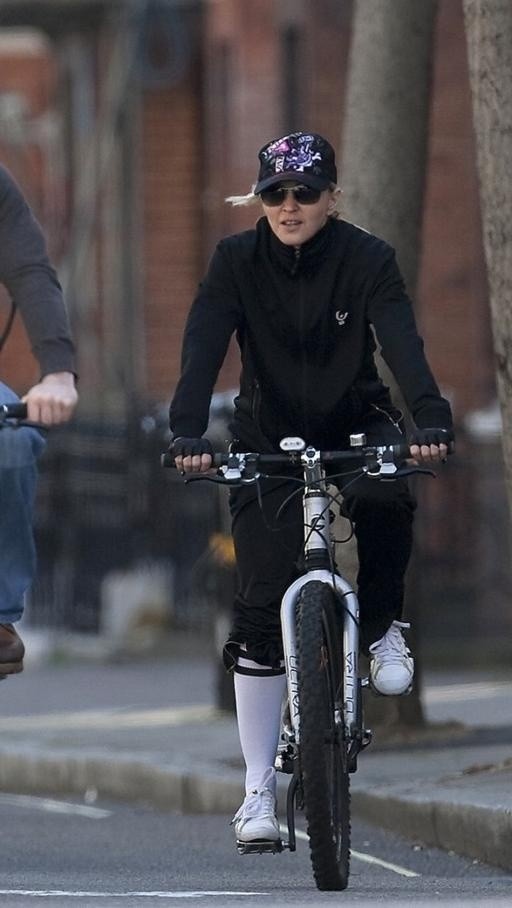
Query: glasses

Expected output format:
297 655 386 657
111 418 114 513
259 182 321 207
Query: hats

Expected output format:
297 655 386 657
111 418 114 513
254 130 338 196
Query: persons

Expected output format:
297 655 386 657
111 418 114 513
1 153 79 676
163 130 455 846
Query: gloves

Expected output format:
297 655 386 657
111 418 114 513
408 426 456 447
168 436 213 460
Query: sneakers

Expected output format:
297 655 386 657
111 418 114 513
234 787 281 842
368 618 414 696
0 622 25 674
